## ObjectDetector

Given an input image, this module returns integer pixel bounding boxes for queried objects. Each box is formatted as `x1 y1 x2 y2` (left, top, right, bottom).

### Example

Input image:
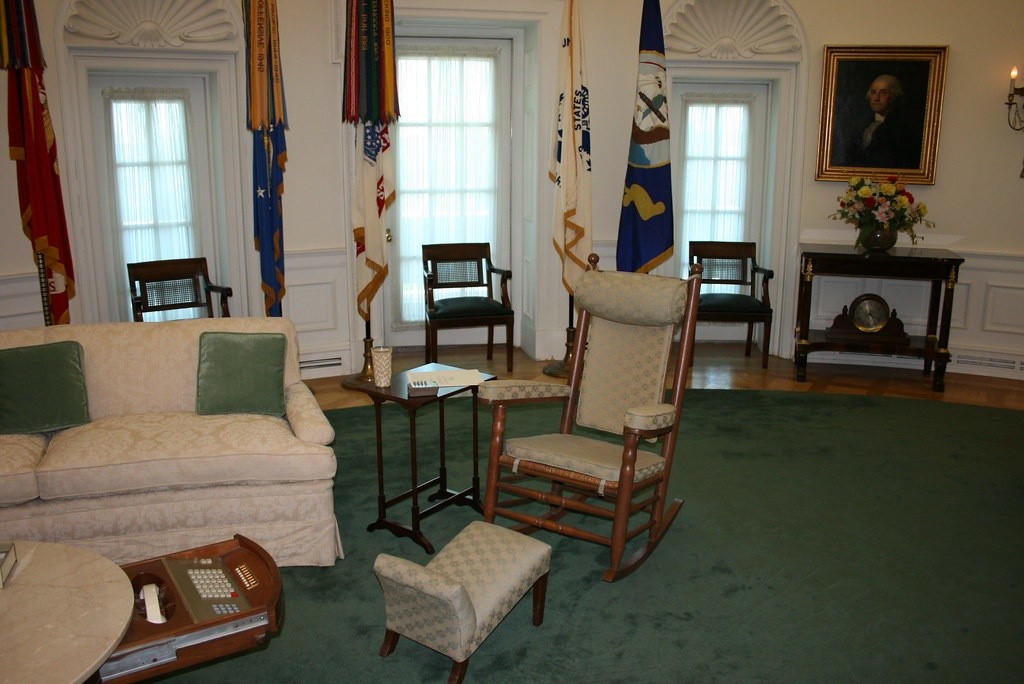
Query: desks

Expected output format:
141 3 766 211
355 362 498 561
0 543 136 684
794 243 965 395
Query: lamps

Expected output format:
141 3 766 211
1003 66 1024 179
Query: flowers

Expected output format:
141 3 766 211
827 168 938 250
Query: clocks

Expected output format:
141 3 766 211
825 295 912 348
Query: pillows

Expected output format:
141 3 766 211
194 332 291 421
0 339 91 436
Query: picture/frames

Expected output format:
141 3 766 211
811 44 951 186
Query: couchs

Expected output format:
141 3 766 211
0 314 346 573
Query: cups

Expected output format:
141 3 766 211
371 346 392 388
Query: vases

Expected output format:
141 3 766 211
861 224 899 252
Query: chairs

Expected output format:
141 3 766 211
127 257 234 324
372 520 552 684
477 253 704 583
688 240 775 369
421 243 516 375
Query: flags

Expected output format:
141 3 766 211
548 0 592 298
253 122 289 317
350 118 397 321
615 0 675 274
7 67 77 327
